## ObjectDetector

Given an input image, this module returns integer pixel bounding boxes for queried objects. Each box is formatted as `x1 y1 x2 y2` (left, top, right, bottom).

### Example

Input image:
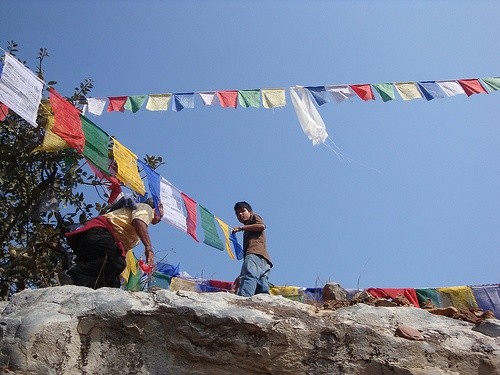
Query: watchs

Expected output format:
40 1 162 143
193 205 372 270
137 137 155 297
239 225 243 231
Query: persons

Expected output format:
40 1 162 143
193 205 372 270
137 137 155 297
230 201 273 297
63 199 164 288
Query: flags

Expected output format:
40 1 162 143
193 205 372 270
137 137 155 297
198 205 224 253
80 77 499 145
214 217 237 261
229 228 247 261
29 87 85 157
139 158 161 216
79 113 110 177
119 247 500 319
0 53 43 129
160 176 188 233
113 135 145 196
181 192 200 244
